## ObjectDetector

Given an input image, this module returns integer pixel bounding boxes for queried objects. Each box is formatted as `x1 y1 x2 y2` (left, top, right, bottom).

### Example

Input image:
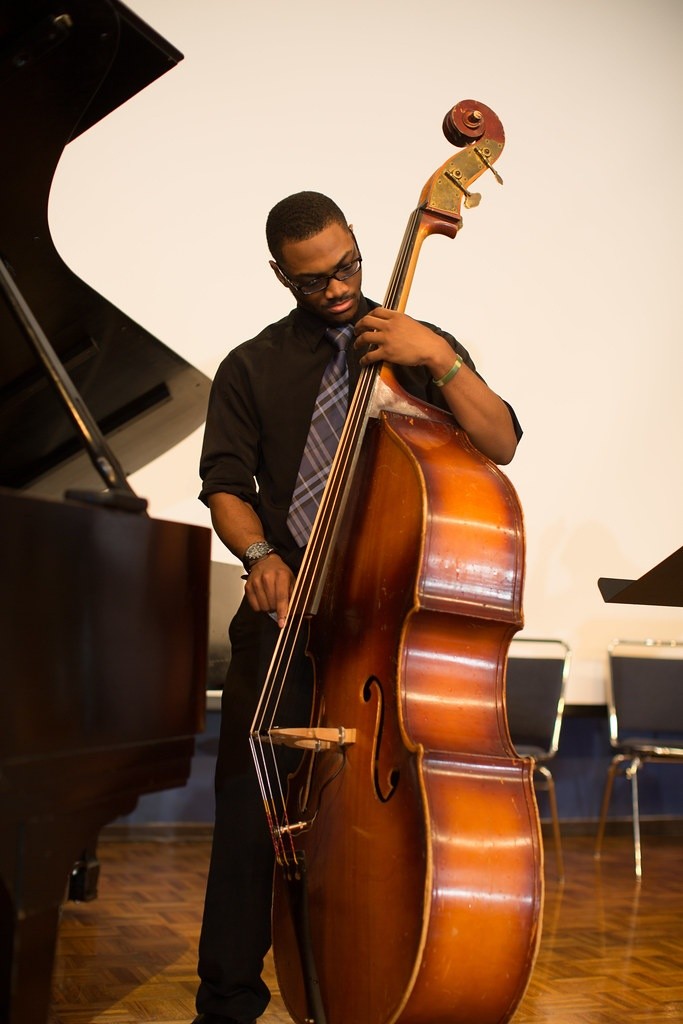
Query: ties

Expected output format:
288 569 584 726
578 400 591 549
285 323 355 547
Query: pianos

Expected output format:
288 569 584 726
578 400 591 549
0 0 220 1024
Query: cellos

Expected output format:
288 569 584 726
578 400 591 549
244 99 546 1024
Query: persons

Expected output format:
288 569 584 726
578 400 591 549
192 190 523 1024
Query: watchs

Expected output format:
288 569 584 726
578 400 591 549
239 542 276 581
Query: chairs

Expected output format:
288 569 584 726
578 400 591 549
594 639 683 884
505 638 570 885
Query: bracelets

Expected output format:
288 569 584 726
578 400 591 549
432 354 463 387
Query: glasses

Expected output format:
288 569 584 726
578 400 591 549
279 229 363 296
